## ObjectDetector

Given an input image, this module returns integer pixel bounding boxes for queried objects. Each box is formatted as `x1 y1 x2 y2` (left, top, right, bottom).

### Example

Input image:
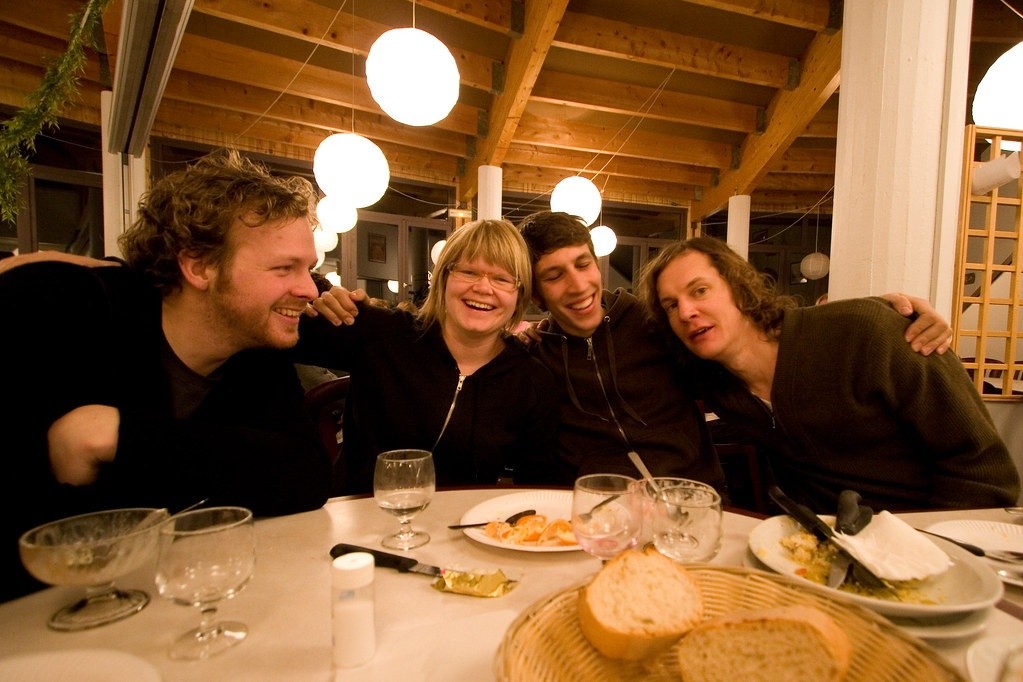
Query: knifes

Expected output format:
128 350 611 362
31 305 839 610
827 490 862 589
330 542 450 578
770 485 905 604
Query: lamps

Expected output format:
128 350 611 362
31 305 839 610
589 211 617 258
313 225 339 252
315 196 359 233
365 0 460 127
799 205 830 280
310 0 390 210
431 239 447 265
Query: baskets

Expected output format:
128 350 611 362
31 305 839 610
494 564 971 682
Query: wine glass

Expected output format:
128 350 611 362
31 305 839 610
158 506 252 660
373 449 436 550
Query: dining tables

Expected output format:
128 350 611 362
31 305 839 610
0 490 1023 682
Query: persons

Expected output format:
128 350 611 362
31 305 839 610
0 151 1021 602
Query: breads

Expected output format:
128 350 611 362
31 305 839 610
577 541 851 682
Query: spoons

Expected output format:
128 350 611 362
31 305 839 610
914 526 985 558
447 509 536 530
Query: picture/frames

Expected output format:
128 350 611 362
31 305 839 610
368 231 387 264
790 261 808 286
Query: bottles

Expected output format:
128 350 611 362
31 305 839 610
332 552 374 666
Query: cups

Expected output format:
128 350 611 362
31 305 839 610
653 487 723 564
573 473 640 560
638 477 713 549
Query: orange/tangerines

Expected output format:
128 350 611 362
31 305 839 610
482 513 579 546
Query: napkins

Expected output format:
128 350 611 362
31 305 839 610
830 509 957 584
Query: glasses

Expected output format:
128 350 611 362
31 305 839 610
448 266 522 293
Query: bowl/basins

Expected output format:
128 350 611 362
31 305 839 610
18 506 170 632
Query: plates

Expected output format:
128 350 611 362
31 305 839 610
749 513 1004 616
927 520 1023 586
966 634 1023 682
884 606 995 639
461 490 580 552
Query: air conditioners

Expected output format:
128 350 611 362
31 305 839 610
550 176 602 226
